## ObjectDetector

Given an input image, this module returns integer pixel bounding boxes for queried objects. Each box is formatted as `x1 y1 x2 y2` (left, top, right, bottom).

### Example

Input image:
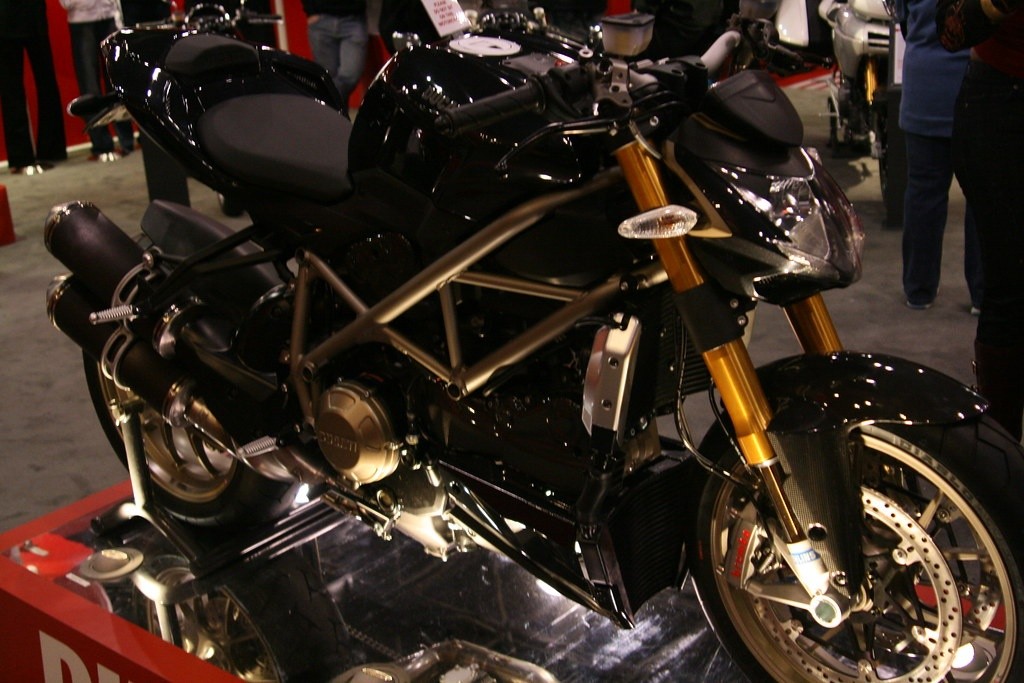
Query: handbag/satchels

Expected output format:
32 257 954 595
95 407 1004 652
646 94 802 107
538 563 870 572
935 0 1022 53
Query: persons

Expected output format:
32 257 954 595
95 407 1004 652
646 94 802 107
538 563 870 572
58 0 135 161
885 0 1024 445
301 0 369 101
0 0 69 175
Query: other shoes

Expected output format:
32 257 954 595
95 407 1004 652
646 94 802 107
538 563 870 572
970 306 982 316
906 289 937 308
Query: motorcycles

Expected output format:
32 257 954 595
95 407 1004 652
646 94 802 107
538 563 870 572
40 0 1024 682
734 0 909 225
